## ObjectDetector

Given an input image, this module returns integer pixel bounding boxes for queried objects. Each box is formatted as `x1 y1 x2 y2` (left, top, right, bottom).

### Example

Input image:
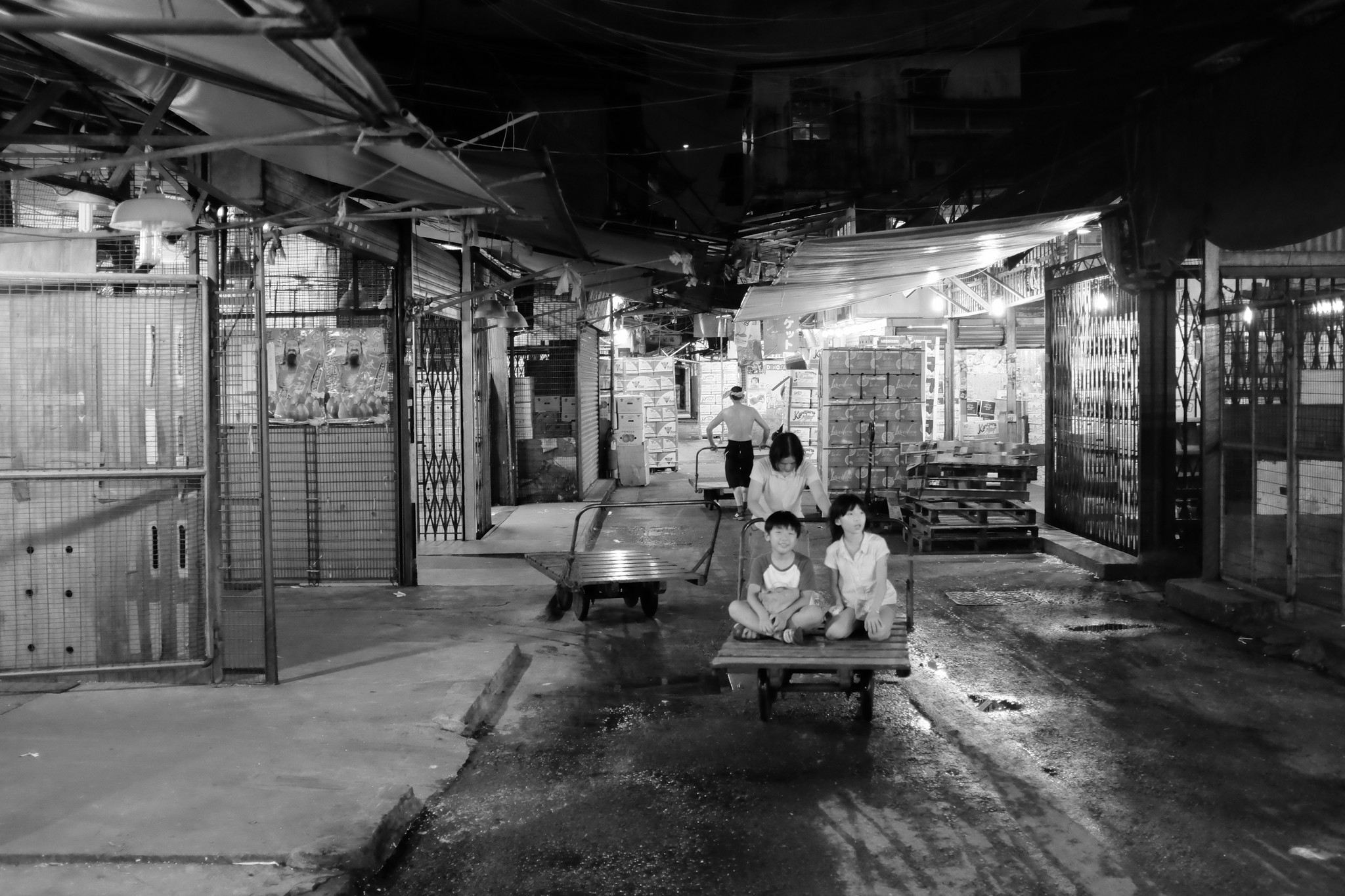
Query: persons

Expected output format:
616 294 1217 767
823 493 897 643
707 386 770 522
323 329 388 418
746 432 833 584
270 329 320 419
728 510 823 645
750 377 760 390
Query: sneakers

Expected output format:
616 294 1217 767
733 511 746 520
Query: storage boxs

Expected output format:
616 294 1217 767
596 335 999 505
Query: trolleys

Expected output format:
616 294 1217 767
709 515 915 723
686 446 772 512
523 498 723 620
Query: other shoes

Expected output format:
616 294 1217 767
733 624 760 640
782 628 803 644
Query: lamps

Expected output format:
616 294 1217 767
225 230 253 280
55 146 196 264
339 278 393 309
473 291 530 328
